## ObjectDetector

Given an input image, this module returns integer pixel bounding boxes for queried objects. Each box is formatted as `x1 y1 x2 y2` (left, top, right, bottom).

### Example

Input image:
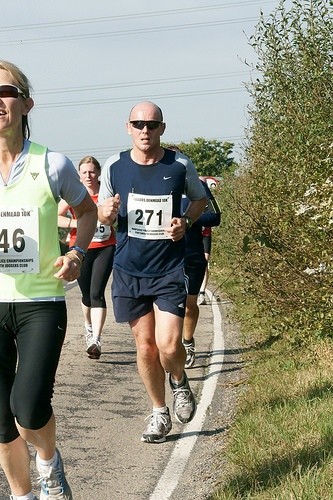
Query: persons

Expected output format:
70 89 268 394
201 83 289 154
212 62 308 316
55 155 117 359
166 146 221 368
98 101 211 444
199 176 212 306
0 59 100 500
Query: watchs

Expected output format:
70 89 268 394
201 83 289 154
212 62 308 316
180 216 192 229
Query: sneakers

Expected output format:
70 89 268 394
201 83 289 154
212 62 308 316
168 369 196 425
87 339 102 359
182 338 196 368
35 446 72 500
140 407 173 443
85 324 93 344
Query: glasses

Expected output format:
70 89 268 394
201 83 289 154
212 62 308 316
0 84 26 99
129 121 162 130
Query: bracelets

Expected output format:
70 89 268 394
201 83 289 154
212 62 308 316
68 218 72 228
66 246 86 261
65 252 82 266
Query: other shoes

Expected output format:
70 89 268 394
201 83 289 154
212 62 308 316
199 295 206 305
